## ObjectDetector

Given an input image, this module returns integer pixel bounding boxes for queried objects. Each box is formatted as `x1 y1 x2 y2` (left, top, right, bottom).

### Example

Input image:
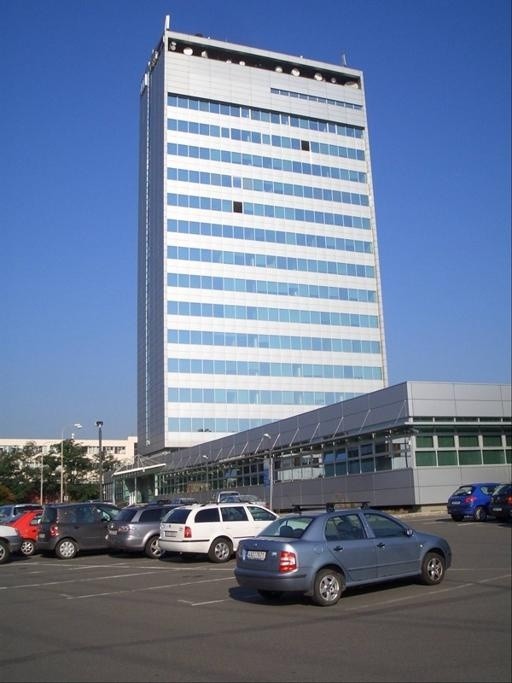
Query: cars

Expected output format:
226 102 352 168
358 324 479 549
232 496 455 605
449 480 512 520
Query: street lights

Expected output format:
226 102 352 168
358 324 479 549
39 442 57 505
59 420 84 502
94 418 107 500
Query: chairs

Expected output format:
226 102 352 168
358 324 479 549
279 525 295 536
336 521 355 539
